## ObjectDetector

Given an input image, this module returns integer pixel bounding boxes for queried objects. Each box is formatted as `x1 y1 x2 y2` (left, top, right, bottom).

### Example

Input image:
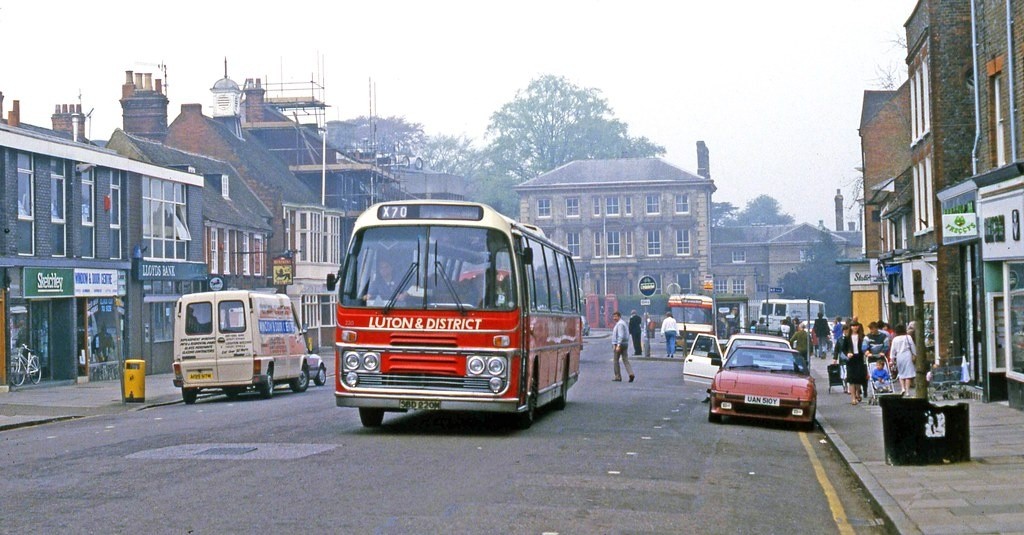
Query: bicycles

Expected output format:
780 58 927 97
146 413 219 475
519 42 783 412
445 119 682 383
11 344 41 387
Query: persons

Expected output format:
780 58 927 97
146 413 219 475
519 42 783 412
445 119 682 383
361 258 405 302
778 316 799 340
660 311 680 358
611 312 634 382
628 310 642 355
749 320 758 334
755 317 768 334
832 316 916 404
790 323 813 363
812 312 830 359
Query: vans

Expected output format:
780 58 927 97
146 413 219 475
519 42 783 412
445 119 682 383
172 290 312 405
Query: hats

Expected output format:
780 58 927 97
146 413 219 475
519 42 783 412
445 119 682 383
868 322 878 328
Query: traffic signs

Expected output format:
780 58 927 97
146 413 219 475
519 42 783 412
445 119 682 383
768 287 784 293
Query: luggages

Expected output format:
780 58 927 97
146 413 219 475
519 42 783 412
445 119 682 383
826 363 844 393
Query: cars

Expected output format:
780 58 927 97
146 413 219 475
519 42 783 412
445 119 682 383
581 315 590 336
682 332 796 384
305 348 326 387
799 321 846 342
706 344 818 432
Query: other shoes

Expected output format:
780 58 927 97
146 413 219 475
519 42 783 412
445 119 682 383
852 400 857 405
629 375 634 382
857 397 861 402
900 389 905 397
905 392 909 397
612 378 621 381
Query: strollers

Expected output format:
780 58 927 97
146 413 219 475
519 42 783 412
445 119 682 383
866 353 895 406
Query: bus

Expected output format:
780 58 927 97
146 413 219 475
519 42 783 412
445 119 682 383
326 200 584 430
714 292 749 344
757 299 826 340
667 293 714 350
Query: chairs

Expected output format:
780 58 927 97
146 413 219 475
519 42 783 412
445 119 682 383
736 355 753 366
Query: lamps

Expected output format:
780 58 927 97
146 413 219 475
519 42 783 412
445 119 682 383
76 162 97 173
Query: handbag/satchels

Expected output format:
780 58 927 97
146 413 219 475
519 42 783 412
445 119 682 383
912 354 916 364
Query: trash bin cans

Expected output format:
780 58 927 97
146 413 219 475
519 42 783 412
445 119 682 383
124 359 145 403
